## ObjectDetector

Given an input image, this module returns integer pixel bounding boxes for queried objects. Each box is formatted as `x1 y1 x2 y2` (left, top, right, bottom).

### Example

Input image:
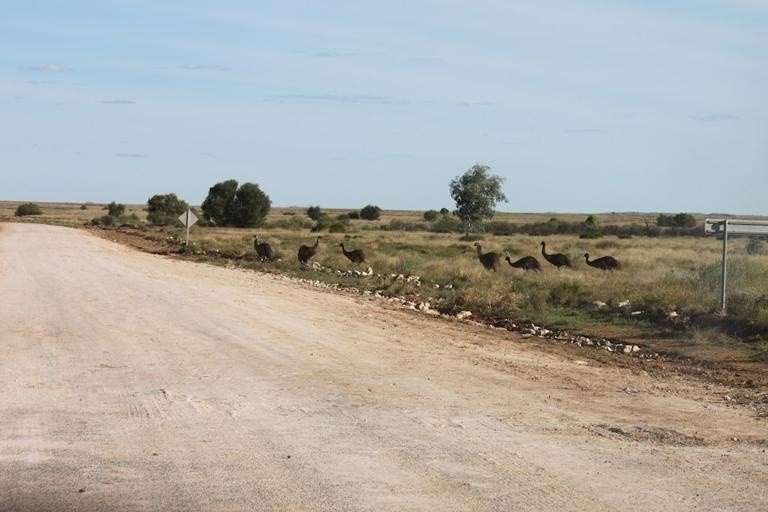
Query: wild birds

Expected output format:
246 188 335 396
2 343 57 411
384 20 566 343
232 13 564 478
297 235 323 272
472 242 499 273
504 256 541 273
583 252 619 270
339 242 365 264
253 234 279 262
540 241 572 270
